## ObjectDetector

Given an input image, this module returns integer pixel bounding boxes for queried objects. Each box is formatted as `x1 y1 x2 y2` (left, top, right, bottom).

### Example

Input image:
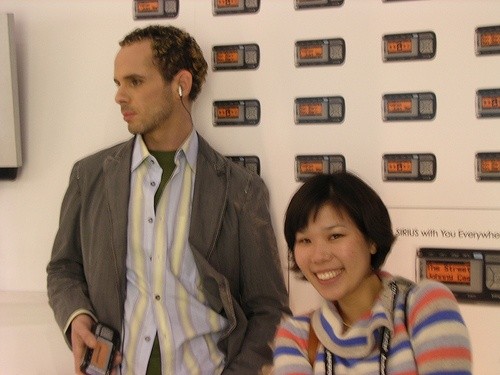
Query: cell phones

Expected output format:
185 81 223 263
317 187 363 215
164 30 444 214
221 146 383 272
80 322 121 375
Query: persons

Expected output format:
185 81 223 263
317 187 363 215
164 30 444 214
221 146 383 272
273 170 474 375
47 25 294 375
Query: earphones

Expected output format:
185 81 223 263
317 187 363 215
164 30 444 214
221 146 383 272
178 85 182 98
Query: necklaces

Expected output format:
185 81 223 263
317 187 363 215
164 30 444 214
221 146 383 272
343 321 352 329
